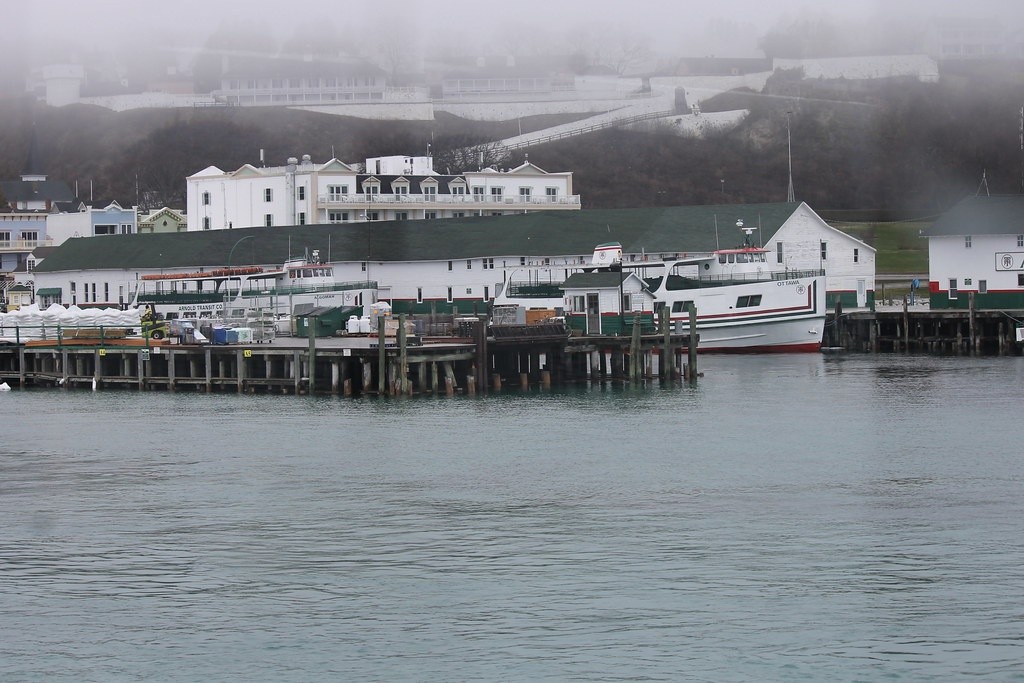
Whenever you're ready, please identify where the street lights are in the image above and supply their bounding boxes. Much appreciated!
[785,111,796,201]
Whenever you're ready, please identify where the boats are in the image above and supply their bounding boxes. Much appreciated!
[493,226,826,356]
[127,257,377,338]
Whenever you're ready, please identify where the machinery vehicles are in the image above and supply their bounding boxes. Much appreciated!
[128,302,166,340]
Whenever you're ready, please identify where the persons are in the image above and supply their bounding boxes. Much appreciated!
[617,251,621,257]
